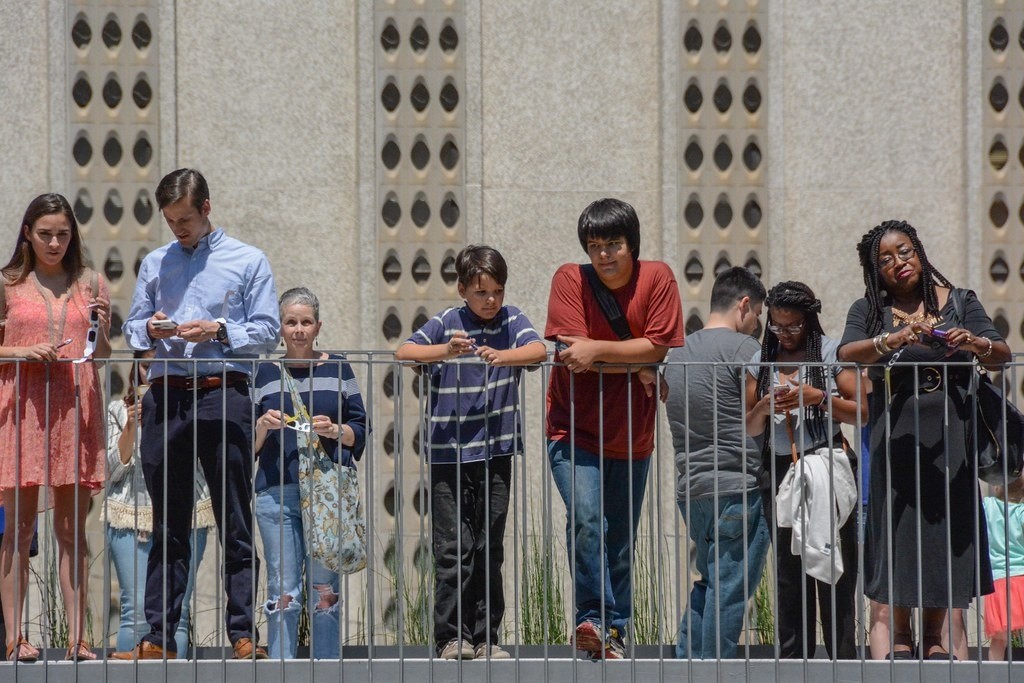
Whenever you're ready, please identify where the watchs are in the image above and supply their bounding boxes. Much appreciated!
[211,321,227,341]
[878,332,893,352]
[815,390,829,409]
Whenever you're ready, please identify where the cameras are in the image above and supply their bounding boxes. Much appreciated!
[921,329,962,351]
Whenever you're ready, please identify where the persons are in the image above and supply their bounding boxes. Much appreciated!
[395,244,548,659]
[0,194,112,662]
[247,287,373,659]
[834,362,1024,661]
[543,198,685,658]
[99,349,217,659]
[742,281,870,659]
[662,267,771,659]
[112,169,281,660]
[843,219,1014,660]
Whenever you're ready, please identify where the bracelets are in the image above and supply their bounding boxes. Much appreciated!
[978,337,992,358]
[333,424,344,439]
[873,335,885,357]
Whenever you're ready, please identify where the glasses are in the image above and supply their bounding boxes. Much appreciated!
[767,318,807,335]
[877,247,917,269]
[72,304,100,364]
[285,405,318,433]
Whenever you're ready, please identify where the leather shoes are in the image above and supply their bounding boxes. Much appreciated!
[108,642,177,660]
[232,637,269,658]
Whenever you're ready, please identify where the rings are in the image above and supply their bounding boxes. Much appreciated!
[966,333,971,343]
[908,334,916,340]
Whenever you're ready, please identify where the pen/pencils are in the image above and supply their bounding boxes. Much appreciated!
[57,339,73,348]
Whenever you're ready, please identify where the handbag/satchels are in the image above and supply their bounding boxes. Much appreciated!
[299,462,367,575]
[964,374,1024,485]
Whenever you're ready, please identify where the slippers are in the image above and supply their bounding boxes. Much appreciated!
[6,637,39,660]
[64,639,96,659]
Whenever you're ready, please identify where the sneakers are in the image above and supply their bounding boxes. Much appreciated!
[474,641,510,659]
[592,629,626,659]
[570,621,611,651]
[441,638,475,659]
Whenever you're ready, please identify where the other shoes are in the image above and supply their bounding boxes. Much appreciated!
[916,644,957,660]
[886,651,913,660]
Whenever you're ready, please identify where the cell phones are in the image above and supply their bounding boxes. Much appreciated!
[151,320,179,331]
[769,384,791,399]
